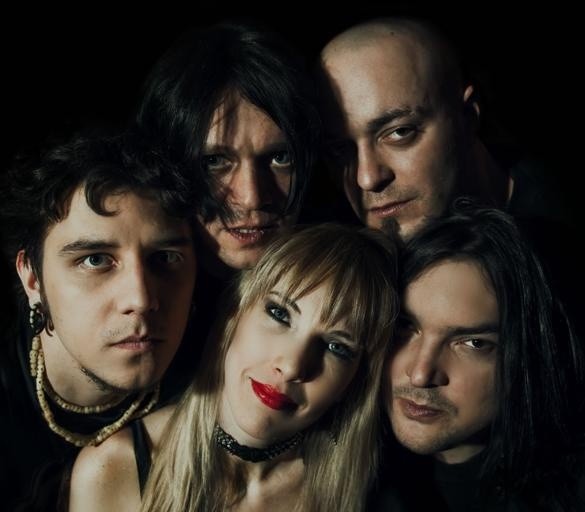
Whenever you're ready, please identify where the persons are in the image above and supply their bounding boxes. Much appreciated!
[128,29,345,270]
[62,213,406,510]
[2,117,238,511]
[369,199,585,511]
[311,16,524,247]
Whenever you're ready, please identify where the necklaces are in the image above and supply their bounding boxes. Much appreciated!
[27,334,162,449]
[207,424,308,462]
